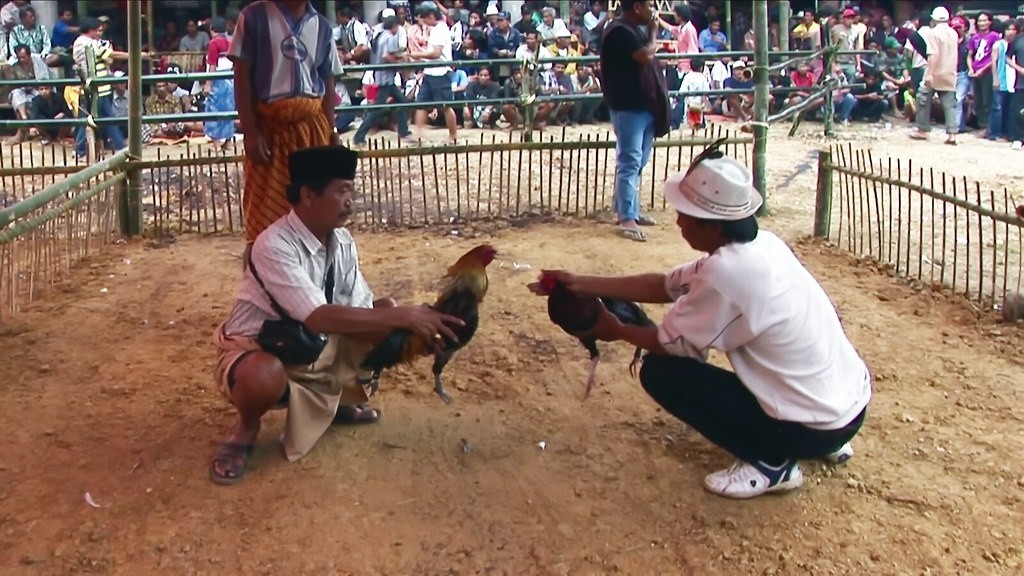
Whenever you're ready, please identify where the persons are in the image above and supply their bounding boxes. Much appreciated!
[602,1,673,243]
[0,0,1024,163]
[524,138,873,500]
[224,0,342,274]
[206,144,468,486]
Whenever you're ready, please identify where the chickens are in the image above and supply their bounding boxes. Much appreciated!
[543,272,657,404]
[360,245,502,406]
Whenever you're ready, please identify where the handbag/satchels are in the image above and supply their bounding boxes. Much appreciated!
[258,318,328,367]
[686,102,703,111]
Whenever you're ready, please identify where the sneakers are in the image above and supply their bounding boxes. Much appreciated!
[823,441,856,464]
[704,457,803,499]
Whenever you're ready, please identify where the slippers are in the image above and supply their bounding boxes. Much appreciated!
[332,404,382,423]
[636,214,658,226]
[209,441,253,484]
[619,224,648,242]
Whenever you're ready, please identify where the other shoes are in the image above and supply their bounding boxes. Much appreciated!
[910,131,1022,150]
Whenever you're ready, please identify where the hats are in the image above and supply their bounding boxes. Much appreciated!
[732,61,746,68]
[664,139,763,221]
[481,1,578,43]
[166,63,182,75]
[287,145,358,180]
[791,5,966,48]
[382,7,396,18]
[113,70,127,78]
